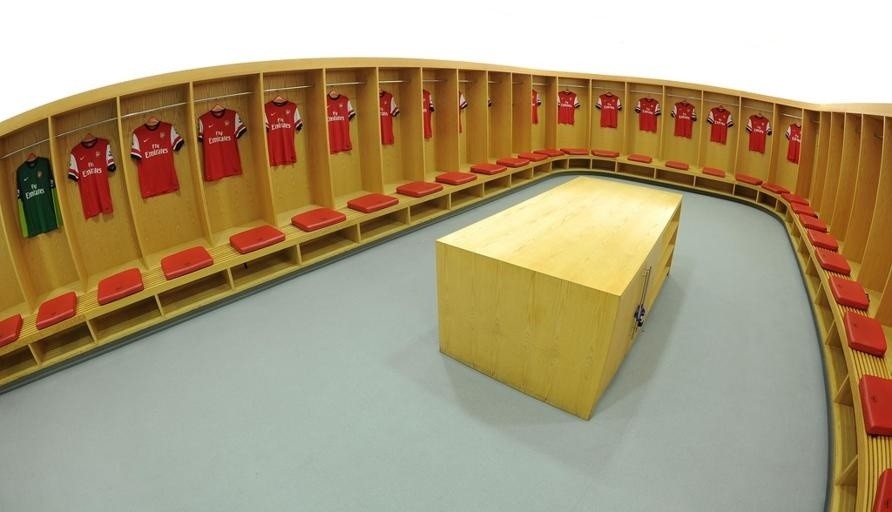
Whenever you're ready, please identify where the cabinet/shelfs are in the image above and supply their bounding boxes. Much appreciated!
[432,175,685,421]
